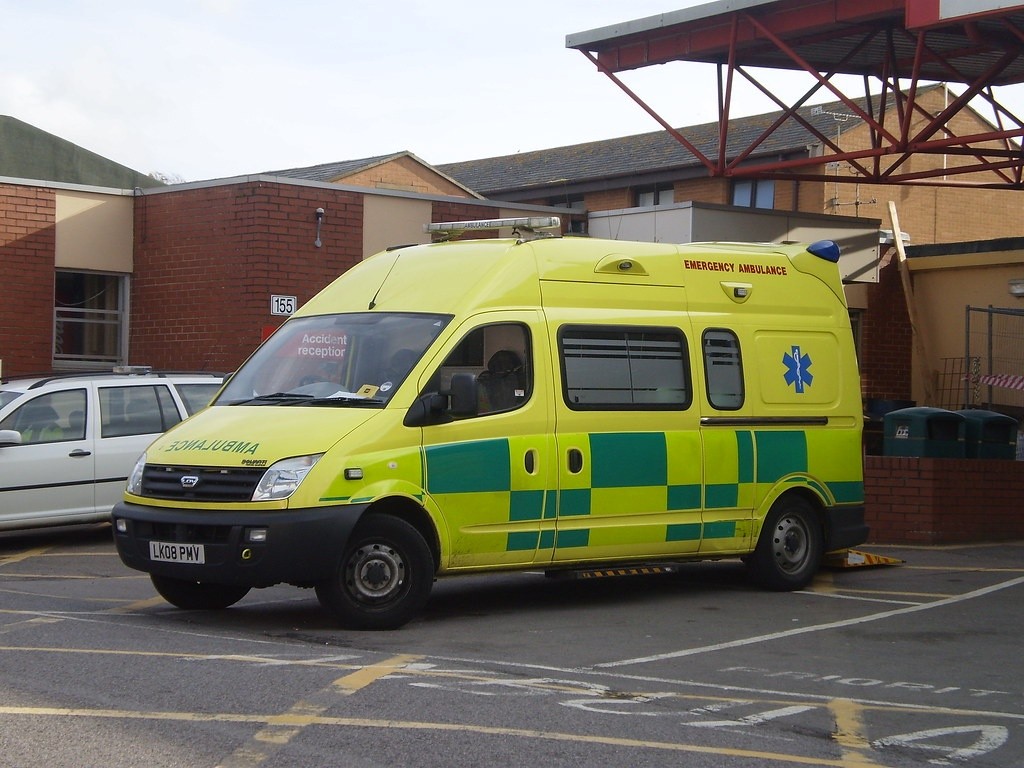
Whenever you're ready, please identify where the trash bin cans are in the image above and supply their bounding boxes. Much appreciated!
[882,407,967,457]
[957,409,1019,459]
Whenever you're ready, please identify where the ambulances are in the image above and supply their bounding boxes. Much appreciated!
[108,216,865,631]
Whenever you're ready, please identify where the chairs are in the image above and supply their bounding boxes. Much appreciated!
[472,350,524,414]
[64,407,84,439]
[376,348,419,399]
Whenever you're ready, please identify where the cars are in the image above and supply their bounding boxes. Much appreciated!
[0,366,260,535]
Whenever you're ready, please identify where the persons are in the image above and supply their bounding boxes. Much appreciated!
[20,402,63,443]
